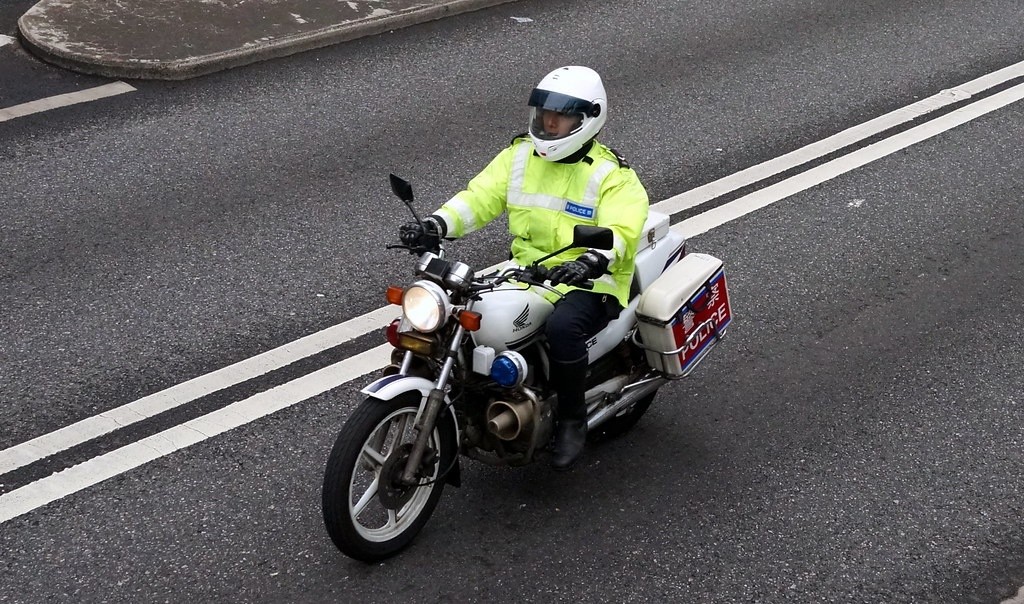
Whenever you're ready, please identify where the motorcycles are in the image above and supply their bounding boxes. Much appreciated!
[319,172,736,566]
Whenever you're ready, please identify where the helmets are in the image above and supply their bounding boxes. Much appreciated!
[528,65,608,162]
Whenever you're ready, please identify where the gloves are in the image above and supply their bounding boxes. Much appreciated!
[400,214,447,246]
[546,250,606,286]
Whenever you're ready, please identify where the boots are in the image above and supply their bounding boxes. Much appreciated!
[550,352,588,470]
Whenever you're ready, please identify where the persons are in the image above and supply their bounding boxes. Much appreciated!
[400,66,650,470]
